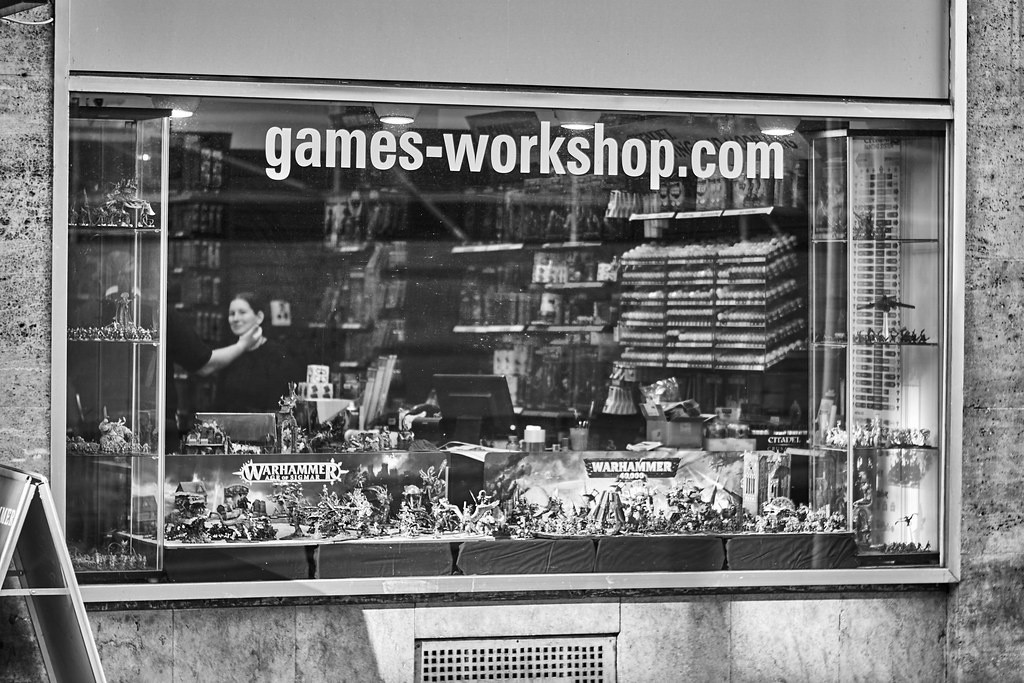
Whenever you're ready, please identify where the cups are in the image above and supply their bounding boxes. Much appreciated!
[522,441,547,453]
[570,428,589,451]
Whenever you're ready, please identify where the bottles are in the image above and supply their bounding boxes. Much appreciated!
[506,436,520,451]
[540,298,556,323]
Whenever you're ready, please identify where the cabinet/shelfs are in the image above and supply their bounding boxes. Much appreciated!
[807,128,943,565]
[171,132,805,372]
[66,107,171,573]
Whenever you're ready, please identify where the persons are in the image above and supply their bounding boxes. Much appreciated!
[68,251,293,451]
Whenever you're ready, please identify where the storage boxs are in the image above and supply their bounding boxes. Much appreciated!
[640,401,807,466]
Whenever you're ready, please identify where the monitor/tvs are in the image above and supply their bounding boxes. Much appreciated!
[433,373,519,445]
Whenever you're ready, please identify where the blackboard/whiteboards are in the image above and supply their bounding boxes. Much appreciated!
[0,463,104,683]
